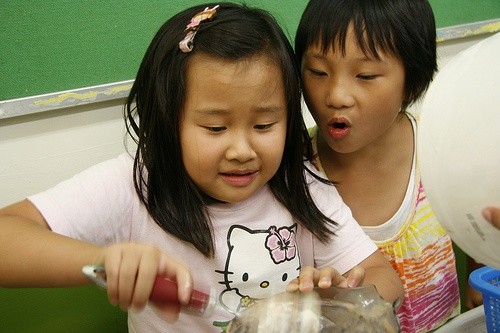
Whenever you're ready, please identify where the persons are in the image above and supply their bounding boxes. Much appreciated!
[1,0,405,333]
[282,0,487,333]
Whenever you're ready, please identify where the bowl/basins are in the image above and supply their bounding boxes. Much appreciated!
[225,282,400,333]
